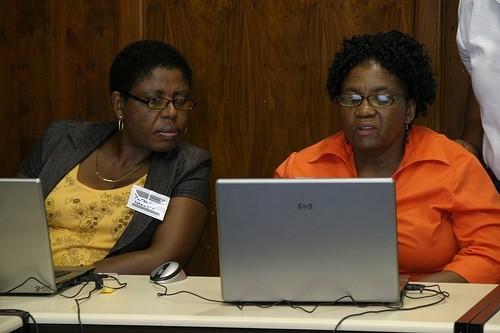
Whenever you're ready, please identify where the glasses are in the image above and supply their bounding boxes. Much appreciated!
[114,90,197,111]
[334,90,413,108]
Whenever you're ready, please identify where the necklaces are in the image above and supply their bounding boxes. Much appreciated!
[96,145,150,184]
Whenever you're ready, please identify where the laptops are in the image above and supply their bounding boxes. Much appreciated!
[214,178,411,303]
[0,178,96,294]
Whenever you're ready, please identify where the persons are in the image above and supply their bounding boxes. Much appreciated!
[456,0,500,191]
[272,30,500,287]
[18,41,213,275]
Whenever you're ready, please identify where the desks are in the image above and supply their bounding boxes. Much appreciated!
[0,273,500,333]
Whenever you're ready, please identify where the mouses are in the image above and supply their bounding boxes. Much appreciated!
[149,261,187,284]
